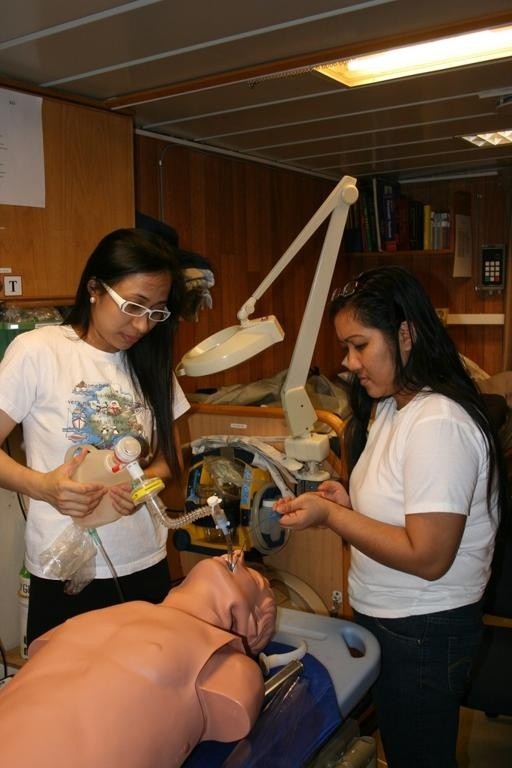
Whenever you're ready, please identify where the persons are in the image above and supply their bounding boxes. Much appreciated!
[0,225,192,656]
[276,267,511,767]
[0,550,279,767]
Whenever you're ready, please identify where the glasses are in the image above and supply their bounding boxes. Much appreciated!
[329,281,360,301]
[102,281,170,322]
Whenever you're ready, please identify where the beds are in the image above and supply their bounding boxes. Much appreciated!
[184,607,391,764]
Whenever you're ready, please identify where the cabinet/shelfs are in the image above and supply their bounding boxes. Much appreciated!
[346,247,508,327]
[0,87,138,314]
